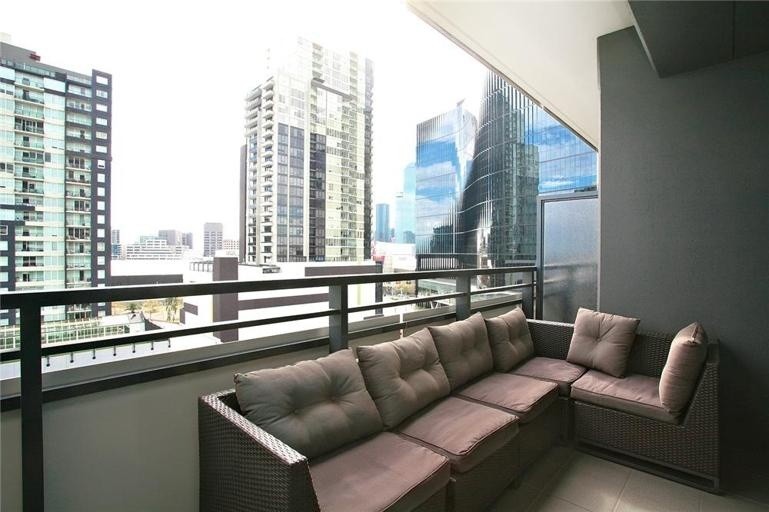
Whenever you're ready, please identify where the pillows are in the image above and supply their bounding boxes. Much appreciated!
[234,345,385,461]
[355,326,453,430]
[565,306,641,379]
[485,306,536,373]
[659,320,710,420]
[429,309,494,393]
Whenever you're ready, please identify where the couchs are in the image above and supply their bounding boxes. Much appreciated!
[198,319,722,512]
